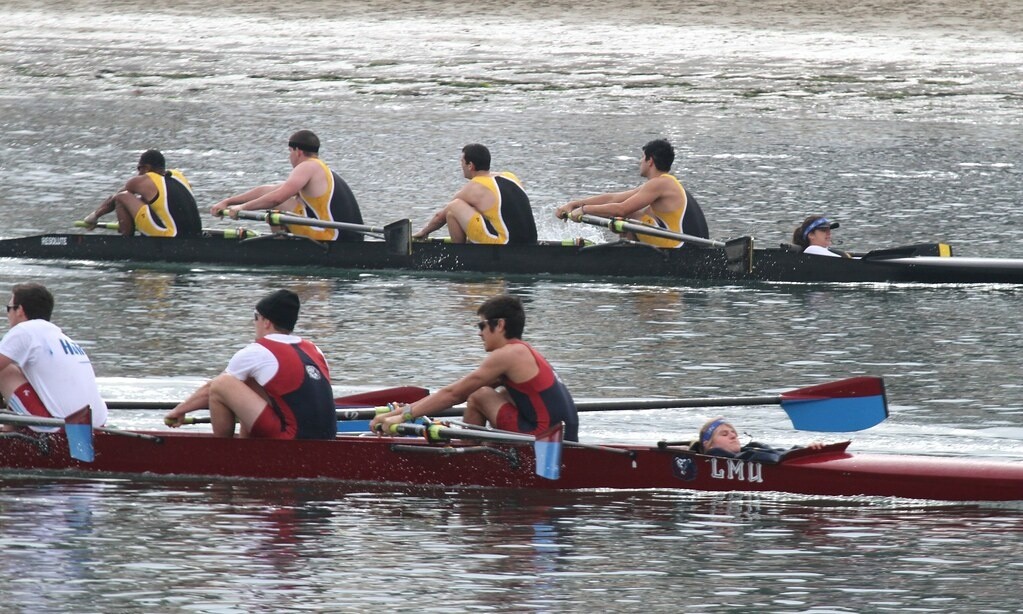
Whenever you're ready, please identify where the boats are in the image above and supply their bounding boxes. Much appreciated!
[0,226,1023,287]
[0,416,1023,500]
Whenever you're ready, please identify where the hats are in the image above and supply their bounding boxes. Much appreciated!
[804,218,840,246]
[256,289,300,330]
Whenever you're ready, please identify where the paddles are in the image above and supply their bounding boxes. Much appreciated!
[778,241,954,261]
[217,209,413,257]
[0,404,95,463]
[414,238,576,249]
[163,376,889,433]
[104,386,430,410]
[74,220,273,240]
[561,212,754,274]
[374,421,566,481]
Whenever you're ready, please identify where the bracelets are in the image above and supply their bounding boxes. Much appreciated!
[582,203,586,214]
[403,408,413,420]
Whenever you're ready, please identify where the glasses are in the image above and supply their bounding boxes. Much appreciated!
[6,305,18,311]
[815,226,834,232]
[137,165,144,170]
[254,311,263,321]
[477,318,505,331]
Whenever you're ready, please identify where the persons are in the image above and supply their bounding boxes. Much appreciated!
[369,295,579,442]
[164,288,337,440]
[210,129,364,241]
[555,140,709,249]
[793,215,840,257]
[84,150,202,237]
[412,143,538,245]
[690,416,825,462]
[0,282,107,433]
